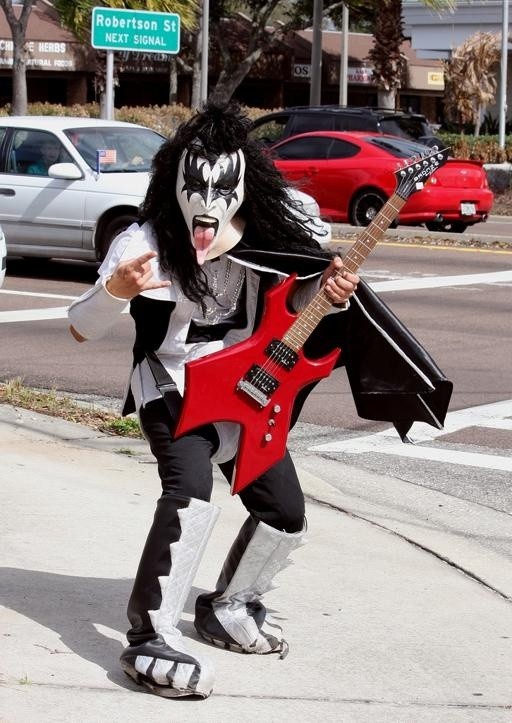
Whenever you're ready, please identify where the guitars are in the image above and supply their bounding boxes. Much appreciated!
[174,146,451,497]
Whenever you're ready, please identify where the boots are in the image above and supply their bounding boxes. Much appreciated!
[191,514,309,658]
[119,492,223,701]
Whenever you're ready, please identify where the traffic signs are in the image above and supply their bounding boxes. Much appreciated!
[91,7,184,56]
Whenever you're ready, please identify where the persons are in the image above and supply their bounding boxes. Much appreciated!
[27,135,62,175]
[67,104,359,699]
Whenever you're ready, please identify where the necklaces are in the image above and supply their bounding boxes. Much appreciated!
[184,261,248,323]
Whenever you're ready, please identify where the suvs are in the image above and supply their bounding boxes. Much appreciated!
[220,97,447,180]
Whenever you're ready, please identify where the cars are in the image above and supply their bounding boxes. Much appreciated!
[1,108,333,289]
[249,126,495,235]
